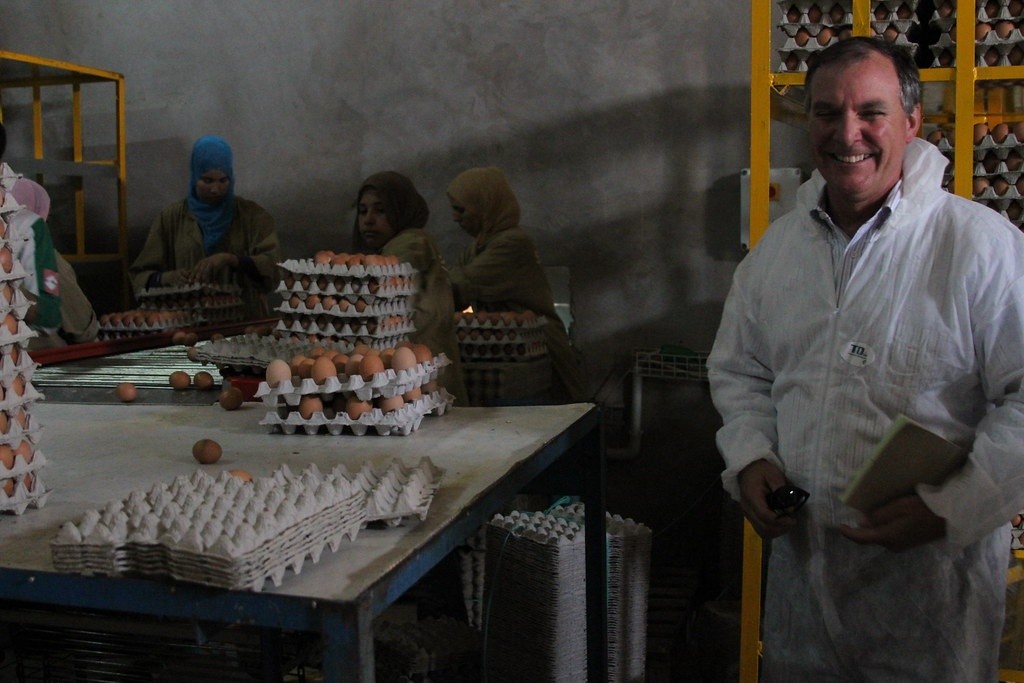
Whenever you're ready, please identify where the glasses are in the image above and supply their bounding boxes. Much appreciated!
[767,486,810,516]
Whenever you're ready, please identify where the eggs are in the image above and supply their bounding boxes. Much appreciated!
[93,282,246,334]
[783,0,1022,229]
[169,347,243,410]
[192,438,222,464]
[117,383,136,401]
[1011,515,1024,550]
[172,326,271,345]
[228,471,252,483]
[264,251,538,419]
[0,185,42,514]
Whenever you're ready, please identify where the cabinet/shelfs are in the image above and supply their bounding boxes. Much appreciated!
[0,50,130,310]
[749,0,1024,251]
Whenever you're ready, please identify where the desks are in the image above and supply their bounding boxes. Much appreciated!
[0,403,609,682]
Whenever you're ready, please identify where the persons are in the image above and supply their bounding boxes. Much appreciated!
[0,122,63,329]
[442,165,579,396]
[11,177,105,350]
[130,135,282,301]
[351,171,472,408]
[706,38,1024,683]
